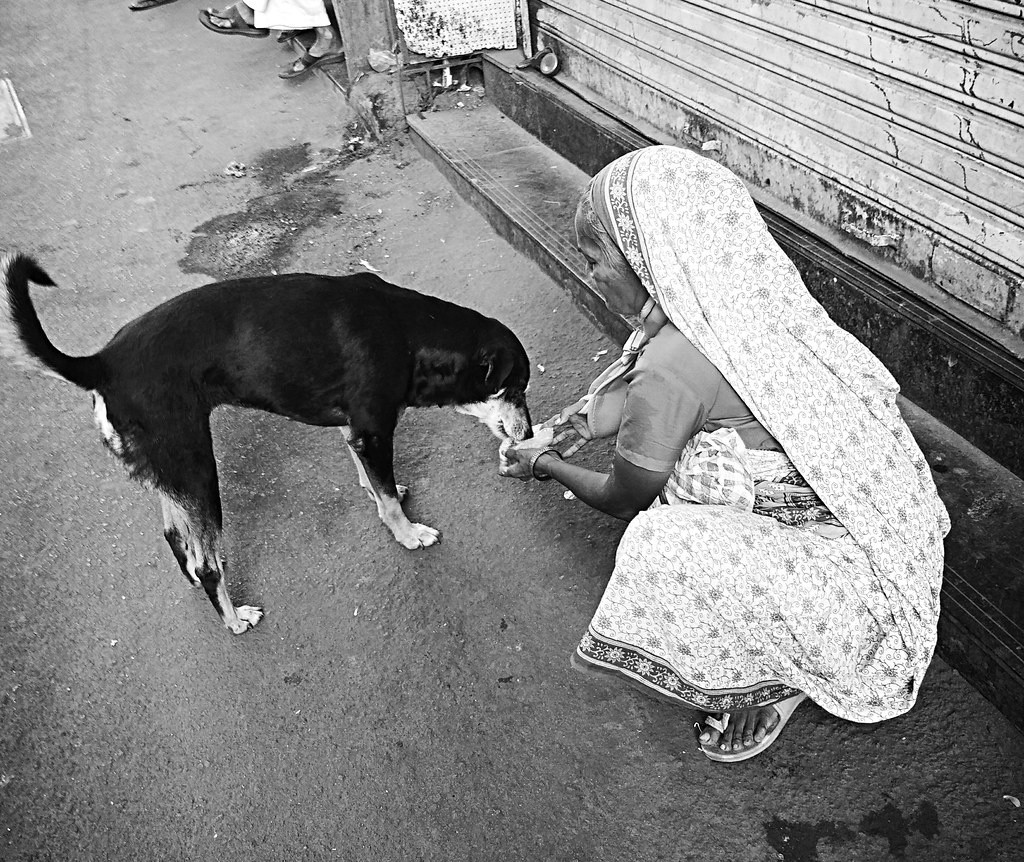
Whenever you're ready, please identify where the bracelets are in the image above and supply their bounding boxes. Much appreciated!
[529,447,564,483]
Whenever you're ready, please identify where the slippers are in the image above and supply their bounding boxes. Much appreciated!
[279,47,346,78]
[198,4,271,38]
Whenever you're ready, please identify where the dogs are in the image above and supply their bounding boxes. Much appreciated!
[0,248,535,639]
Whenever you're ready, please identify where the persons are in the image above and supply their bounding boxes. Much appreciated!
[484,145,951,768]
[128,0,345,81]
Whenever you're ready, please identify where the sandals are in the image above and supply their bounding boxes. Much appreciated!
[698,693,810,763]
